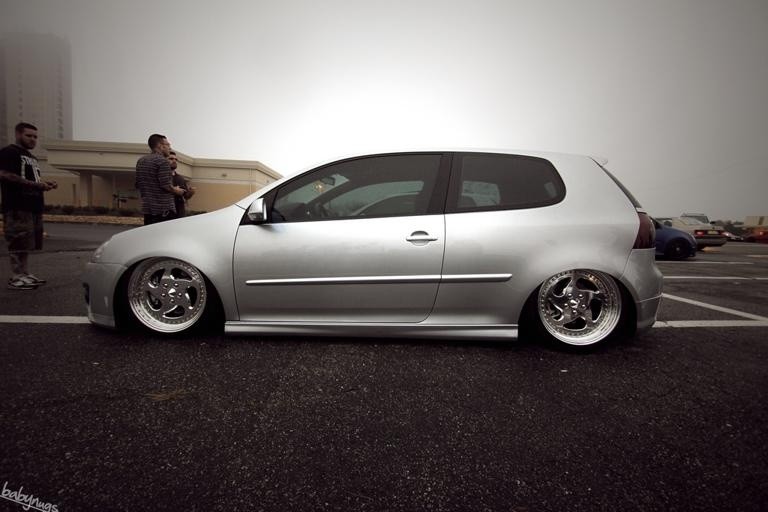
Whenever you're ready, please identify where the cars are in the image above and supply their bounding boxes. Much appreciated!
[80,146,665,355]
[647,211,768,261]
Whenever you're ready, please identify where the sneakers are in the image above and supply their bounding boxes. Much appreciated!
[7,272,48,291]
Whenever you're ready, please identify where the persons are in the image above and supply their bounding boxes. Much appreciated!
[164,152,195,218]
[135,134,185,224]
[0,123,57,290]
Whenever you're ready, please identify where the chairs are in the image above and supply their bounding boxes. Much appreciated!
[409,174,552,212]
